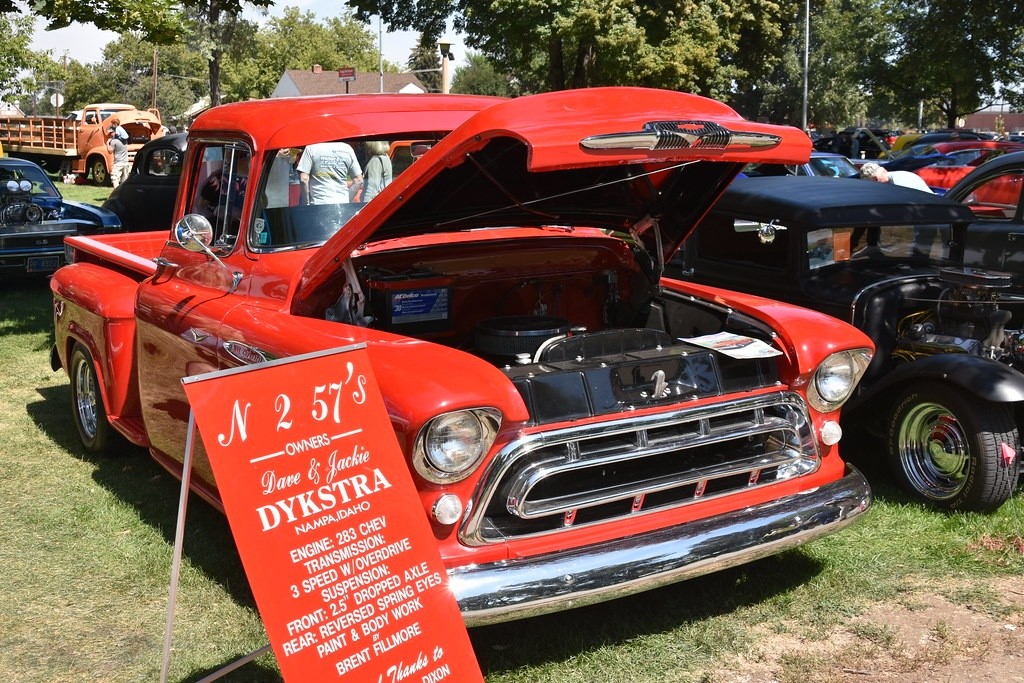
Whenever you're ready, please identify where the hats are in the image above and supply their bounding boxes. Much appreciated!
[860,162,879,179]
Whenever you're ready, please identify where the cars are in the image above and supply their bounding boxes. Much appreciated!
[0,127,1024,511]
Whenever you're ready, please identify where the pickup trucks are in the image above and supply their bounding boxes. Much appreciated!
[47,85,876,630]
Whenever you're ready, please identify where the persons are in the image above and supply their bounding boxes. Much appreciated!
[297,142,364,205]
[359,141,393,203]
[861,162,936,195]
[106,127,129,188]
[110,118,129,140]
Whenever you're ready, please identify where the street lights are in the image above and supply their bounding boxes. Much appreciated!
[435,41,455,92]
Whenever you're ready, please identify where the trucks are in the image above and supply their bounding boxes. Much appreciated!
[0,103,166,186]
